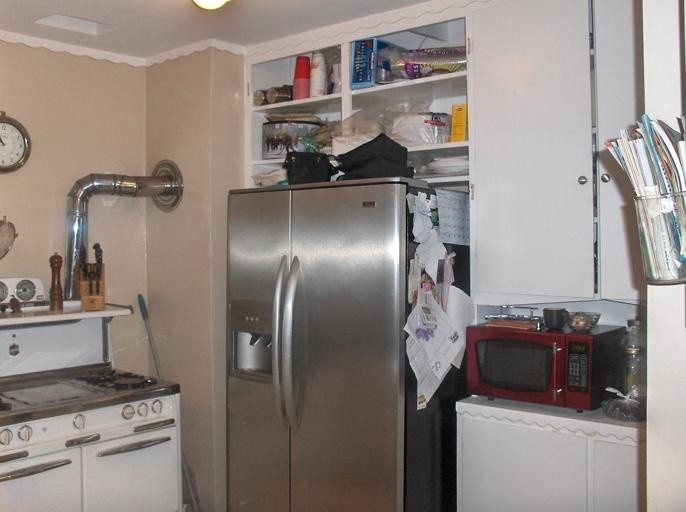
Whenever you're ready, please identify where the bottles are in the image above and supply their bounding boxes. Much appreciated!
[620,343,647,402]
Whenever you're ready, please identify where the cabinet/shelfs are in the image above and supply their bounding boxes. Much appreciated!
[470,0,643,319]
[249,15,468,189]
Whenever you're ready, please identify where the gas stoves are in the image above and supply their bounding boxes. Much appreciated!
[0,360,180,426]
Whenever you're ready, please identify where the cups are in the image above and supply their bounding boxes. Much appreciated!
[543,306,570,330]
[292,52,327,104]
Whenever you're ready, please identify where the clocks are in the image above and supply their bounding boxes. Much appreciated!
[1,111,31,172]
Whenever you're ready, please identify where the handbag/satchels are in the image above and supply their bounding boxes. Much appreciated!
[281,144,339,185]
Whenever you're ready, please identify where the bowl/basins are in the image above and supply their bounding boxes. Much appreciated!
[566,310,602,333]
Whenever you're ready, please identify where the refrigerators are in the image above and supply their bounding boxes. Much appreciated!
[224,176,469,511]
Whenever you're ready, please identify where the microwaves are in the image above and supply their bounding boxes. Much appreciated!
[463,313,628,412]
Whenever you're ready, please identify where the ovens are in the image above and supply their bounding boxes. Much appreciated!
[0,394,182,512]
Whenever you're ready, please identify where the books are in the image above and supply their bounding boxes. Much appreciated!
[603,112,686,284]
